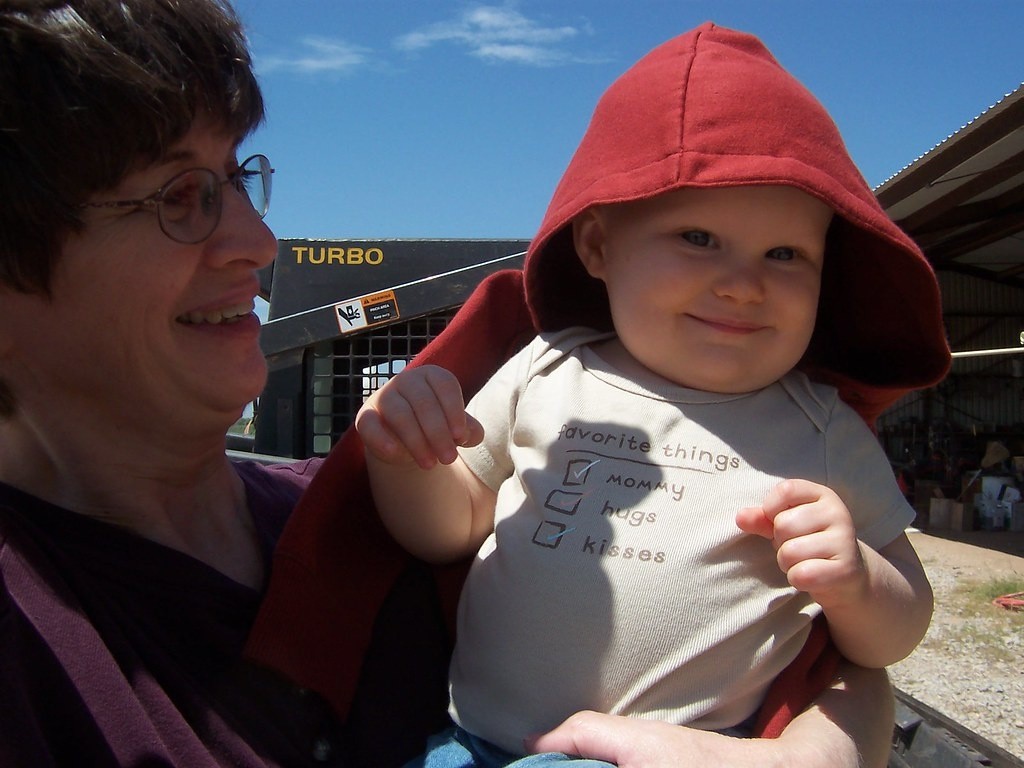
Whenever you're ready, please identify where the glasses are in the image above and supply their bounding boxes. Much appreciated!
[76,154,277,243]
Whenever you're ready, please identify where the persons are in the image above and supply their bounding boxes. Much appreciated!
[0,0,894,768]
[356,22,952,768]
